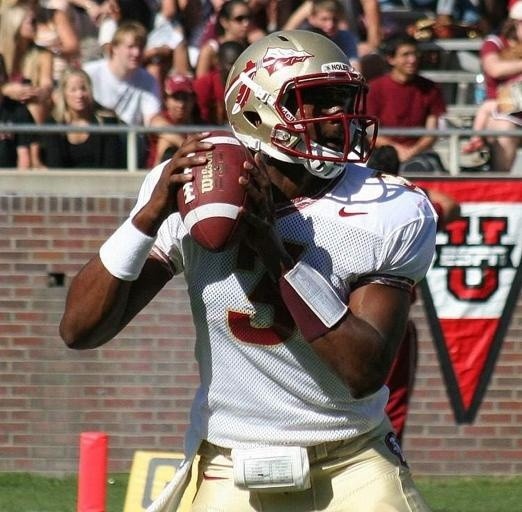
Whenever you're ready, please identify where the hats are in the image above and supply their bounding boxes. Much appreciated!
[162,74,197,96]
[506,1,522,23]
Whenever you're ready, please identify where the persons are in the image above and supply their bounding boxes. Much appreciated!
[60,30,439,511]
[366,145,454,452]
[0,0,522,174]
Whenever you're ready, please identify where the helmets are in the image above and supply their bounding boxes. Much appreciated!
[220,26,367,157]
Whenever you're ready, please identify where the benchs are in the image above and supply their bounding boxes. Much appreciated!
[351,1,522,176]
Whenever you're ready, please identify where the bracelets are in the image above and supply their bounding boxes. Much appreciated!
[275,259,349,344]
[99,217,157,282]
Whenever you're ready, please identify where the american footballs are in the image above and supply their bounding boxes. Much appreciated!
[178,130,254,252]
